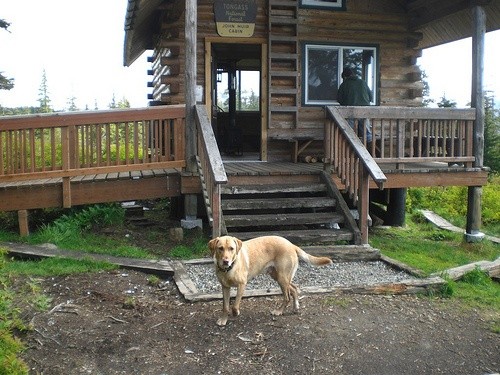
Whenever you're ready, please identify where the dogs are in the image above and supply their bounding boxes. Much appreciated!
[207,235,332,326]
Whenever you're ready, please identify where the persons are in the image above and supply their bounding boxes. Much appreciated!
[337,69,372,153]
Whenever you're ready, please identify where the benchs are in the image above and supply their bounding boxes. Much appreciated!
[270,132,397,164]
